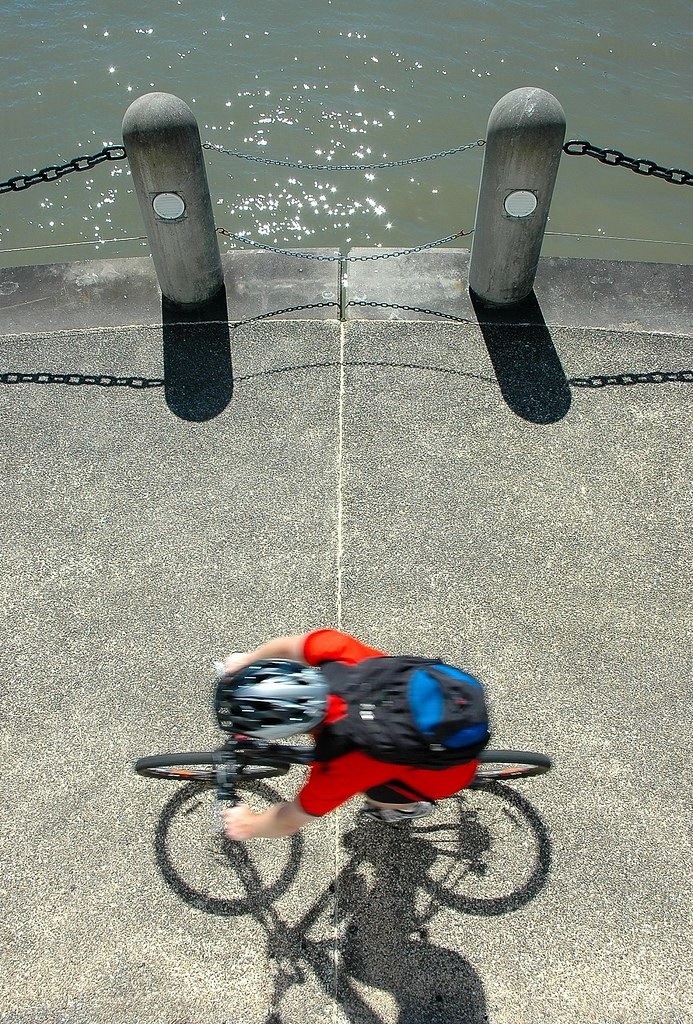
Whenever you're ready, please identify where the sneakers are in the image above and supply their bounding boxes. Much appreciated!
[366,800,433,822]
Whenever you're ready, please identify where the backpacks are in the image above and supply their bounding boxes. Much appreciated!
[314,656,490,770]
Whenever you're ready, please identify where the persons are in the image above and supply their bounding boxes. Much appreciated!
[220,631,482,841]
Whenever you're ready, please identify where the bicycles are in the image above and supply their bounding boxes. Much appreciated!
[131,649,553,826]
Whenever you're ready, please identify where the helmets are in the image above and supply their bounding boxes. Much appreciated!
[215,658,329,740]
